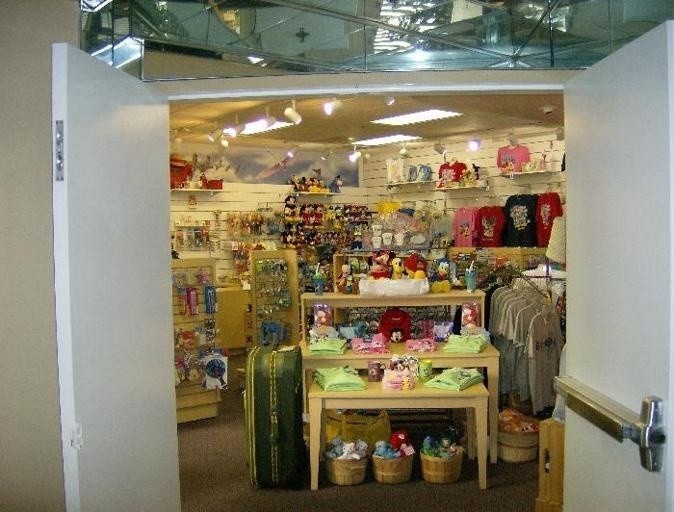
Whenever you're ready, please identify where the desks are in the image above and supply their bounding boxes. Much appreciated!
[333,252,457,326]
[301,289,487,337]
[299,334,501,467]
[307,371,490,491]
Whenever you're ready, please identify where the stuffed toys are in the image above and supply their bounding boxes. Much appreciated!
[374,429,416,457]
[462,307,476,328]
[324,438,369,461]
[283,175,368,246]
[316,306,329,327]
[423,436,457,458]
[500,409,539,432]
[337,249,452,294]
[390,328,403,343]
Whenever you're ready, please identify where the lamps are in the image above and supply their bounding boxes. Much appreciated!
[283,99,303,125]
[209,123,224,143]
[349,145,361,163]
[324,97,344,115]
[257,105,276,129]
[434,143,447,156]
[221,139,230,147]
[230,113,246,138]
[287,145,298,158]
[400,150,409,158]
[383,96,395,107]
[468,139,481,151]
[320,149,331,160]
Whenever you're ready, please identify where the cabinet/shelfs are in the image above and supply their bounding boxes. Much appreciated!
[172,257,222,423]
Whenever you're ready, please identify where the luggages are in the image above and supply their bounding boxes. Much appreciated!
[243,341,303,486]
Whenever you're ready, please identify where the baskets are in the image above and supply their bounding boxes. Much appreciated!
[371,452,413,484]
[324,452,368,486]
[419,446,464,484]
[497,414,542,464]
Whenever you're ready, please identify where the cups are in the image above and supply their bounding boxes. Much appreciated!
[465,271,477,292]
[368,360,386,381]
[419,359,432,381]
[313,279,323,296]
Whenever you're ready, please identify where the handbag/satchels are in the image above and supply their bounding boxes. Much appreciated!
[304,409,391,452]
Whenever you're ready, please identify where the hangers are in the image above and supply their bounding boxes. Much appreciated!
[509,274,554,316]
